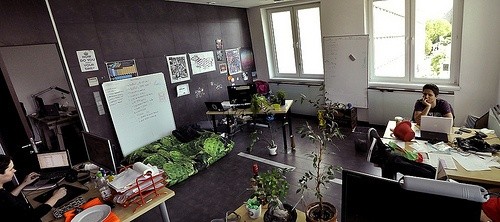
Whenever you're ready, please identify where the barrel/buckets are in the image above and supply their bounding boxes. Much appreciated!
[393,122,415,142]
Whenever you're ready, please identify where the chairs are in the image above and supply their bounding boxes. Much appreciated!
[254,80,272,141]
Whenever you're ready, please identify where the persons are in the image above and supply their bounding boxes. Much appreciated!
[367,128,500,222]
[414,84,453,127]
[0,154,67,222]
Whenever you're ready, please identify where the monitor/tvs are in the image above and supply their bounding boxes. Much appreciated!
[82,131,117,177]
[341,169,482,222]
[227,85,256,104]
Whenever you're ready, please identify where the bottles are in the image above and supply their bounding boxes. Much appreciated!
[252,164,266,203]
[96,172,115,209]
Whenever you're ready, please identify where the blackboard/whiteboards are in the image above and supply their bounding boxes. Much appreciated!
[323,34,369,109]
[101,72,176,157]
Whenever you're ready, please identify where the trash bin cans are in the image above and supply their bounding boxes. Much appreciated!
[352,126,369,146]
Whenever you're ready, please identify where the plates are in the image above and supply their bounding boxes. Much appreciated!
[69,204,111,222]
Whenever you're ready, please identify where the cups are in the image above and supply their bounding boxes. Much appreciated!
[246,203,261,219]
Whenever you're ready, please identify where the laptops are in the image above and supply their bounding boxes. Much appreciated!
[420,116,453,134]
[204,101,230,111]
[23,149,72,190]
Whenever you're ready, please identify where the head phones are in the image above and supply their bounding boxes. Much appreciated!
[64,168,90,183]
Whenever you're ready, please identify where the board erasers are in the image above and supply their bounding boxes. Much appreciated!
[349,55,355,61]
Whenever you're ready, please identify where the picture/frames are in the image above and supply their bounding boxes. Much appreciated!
[225,47,243,76]
[218,63,228,75]
[188,50,216,74]
[165,53,191,84]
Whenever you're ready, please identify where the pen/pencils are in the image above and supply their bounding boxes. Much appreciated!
[56,183,59,190]
[417,100,423,102]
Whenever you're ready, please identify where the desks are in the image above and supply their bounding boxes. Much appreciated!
[29,107,80,152]
[205,99,294,150]
[228,202,306,222]
[15,162,174,222]
[375,119,500,185]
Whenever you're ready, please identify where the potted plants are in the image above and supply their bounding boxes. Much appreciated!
[245,197,261,219]
[253,170,297,222]
[298,83,348,222]
[246,111,278,156]
[277,90,287,106]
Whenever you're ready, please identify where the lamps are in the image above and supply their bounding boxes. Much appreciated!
[29,86,70,105]
